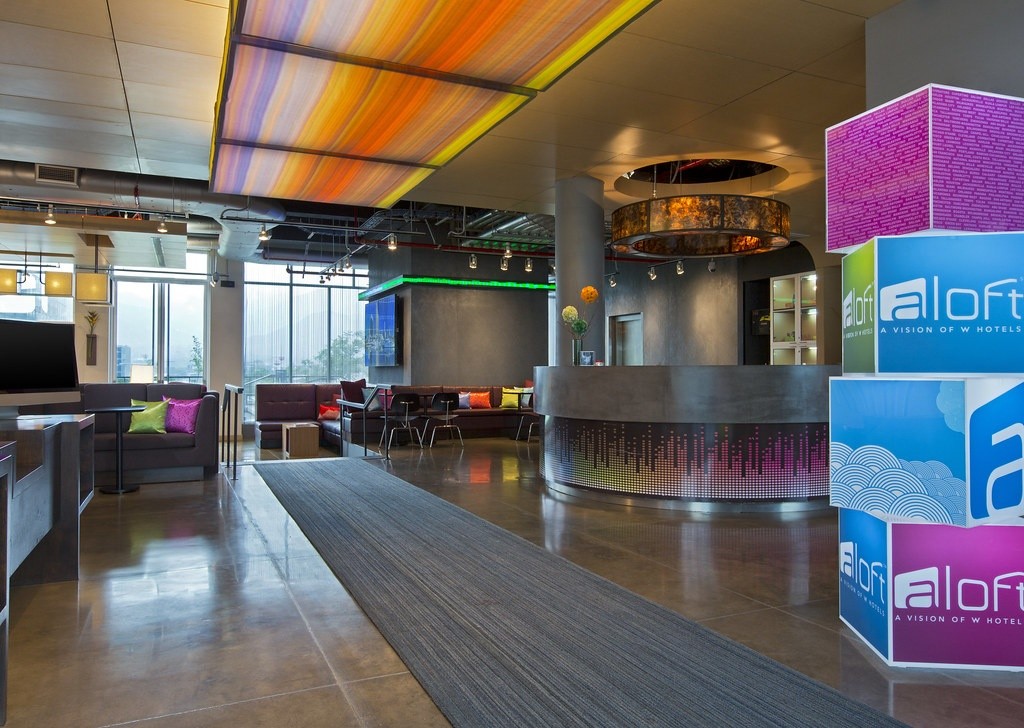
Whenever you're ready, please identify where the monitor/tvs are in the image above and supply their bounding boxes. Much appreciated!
[0,319,81,420]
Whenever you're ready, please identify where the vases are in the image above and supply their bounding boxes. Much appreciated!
[572,339,583,366]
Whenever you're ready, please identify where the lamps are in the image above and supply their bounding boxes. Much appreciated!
[45,206,56,225]
[84,406,144,494]
[469,241,556,273]
[320,255,351,283]
[387,233,398,251]
[0,231,114,306]
[608,162,791,286]
[209,272,220,288]
[157,215,168,233]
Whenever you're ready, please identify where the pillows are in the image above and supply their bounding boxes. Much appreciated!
[129,396,202,434]
[317,380,535,420]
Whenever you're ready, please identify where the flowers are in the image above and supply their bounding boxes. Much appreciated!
[562,286,599,338]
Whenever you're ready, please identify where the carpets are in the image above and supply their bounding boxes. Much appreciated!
[252,457,915,728]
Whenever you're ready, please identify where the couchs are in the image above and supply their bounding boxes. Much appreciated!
[18,383,221,486]
[255,382,372,448]
[391,384,539,439]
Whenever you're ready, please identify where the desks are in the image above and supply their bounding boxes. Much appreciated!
[0,413,97,727]
[503,392,533,438]
[379,394,435,446]
[282,424,320,459]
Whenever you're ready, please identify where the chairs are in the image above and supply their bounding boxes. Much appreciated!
[378,392,423,449]
[516,412,540,443]
[419,393,464,448]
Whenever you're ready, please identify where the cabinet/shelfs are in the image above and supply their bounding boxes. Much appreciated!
[769,271,817,367]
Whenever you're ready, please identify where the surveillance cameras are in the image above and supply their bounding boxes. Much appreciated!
[213,272,220,282]
[708,262,717,272]
